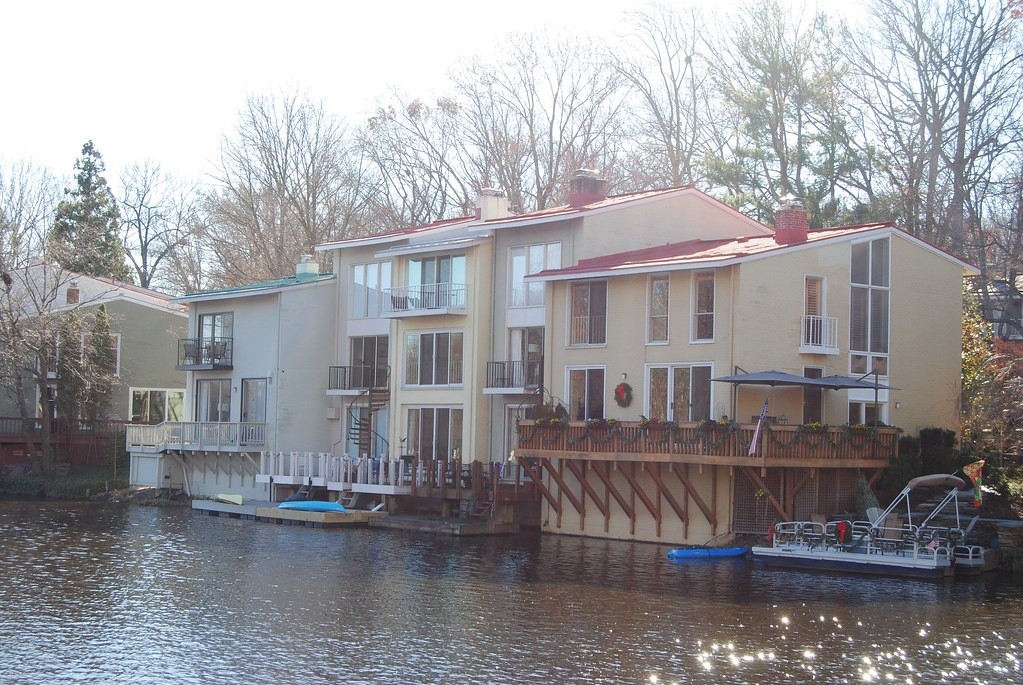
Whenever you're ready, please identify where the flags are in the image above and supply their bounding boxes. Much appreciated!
[749,400,768,456]
[928,534,939,549]
[964,461,985,508]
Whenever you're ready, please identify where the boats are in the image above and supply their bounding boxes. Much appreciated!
[750,473,986,582]
[666,546,750,559]
[216,494,244,505]
[278,501,348,513]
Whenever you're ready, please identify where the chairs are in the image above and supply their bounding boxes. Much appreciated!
[207,342,228,364]
[182,344,201,362]
[874,518,906,558]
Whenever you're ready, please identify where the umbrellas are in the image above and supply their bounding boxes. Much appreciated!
[707,365,902,427]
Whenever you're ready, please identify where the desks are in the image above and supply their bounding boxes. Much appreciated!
[874,538,906,554]
